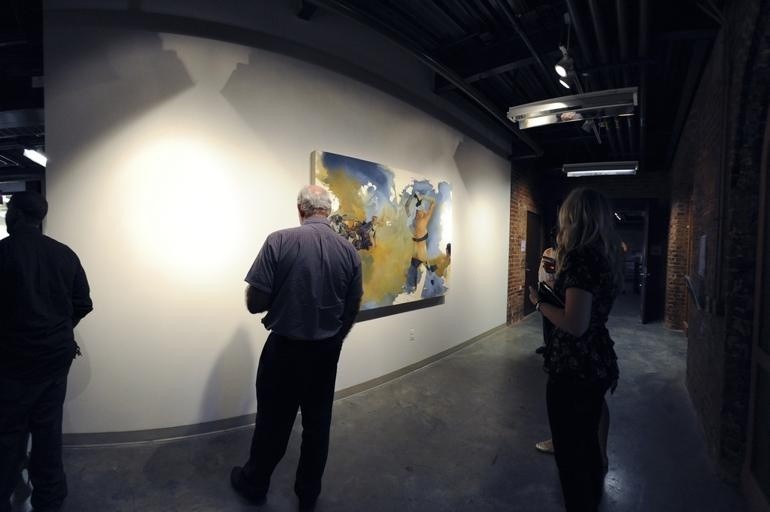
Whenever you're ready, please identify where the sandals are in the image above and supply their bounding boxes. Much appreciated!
[536,439,555,454]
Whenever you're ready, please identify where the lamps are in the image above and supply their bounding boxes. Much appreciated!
[509,47,640,130]
[561,159,640,180]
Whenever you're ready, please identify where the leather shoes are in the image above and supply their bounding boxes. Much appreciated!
[231,466,266,505]
[536,345,546,353]
[295,481,316,512]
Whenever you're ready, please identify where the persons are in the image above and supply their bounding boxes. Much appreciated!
[529,188,620,512]
[0,192,93,512]
[230,184,363,512]
[329,193,451,278]
